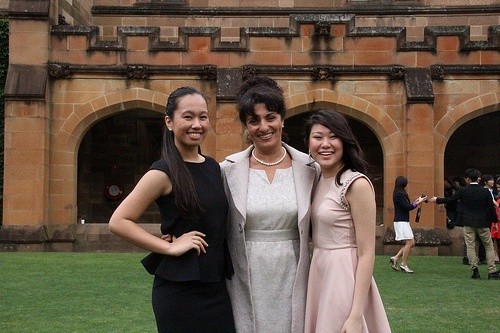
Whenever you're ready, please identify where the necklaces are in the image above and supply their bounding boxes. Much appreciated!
[252,146,286,166]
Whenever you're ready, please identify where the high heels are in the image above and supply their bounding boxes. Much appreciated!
[399,263,413,273]
[388,256,399,271]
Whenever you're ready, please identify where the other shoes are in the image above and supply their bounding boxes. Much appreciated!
[488,271,500,279]
[471,268,481,280]
[462,256,471,265]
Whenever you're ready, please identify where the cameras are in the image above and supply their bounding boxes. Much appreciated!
[421,193,429,203]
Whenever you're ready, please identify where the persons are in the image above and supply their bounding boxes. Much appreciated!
[387,176,428,273]
[161,76,337,333]
[429,169,500,279]
[109,86,236,333]
[304,110,390,333]
